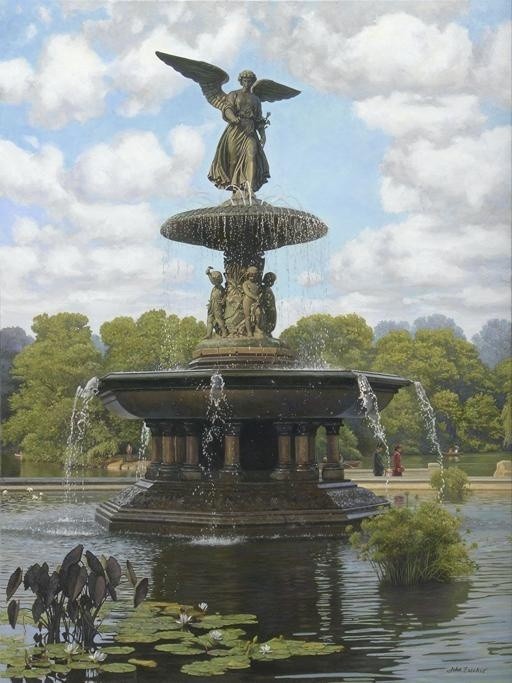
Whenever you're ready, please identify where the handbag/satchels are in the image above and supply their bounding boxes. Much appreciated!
[396,466,404,472]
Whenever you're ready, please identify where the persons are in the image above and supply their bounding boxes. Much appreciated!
[242,265,264,336]
[207,69,271,204]
[261,271,277,337]
[201,266,228,339]
[392,444,405,475]
[373,441,386,476]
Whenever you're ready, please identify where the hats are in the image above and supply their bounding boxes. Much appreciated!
[395,444,402,448]
